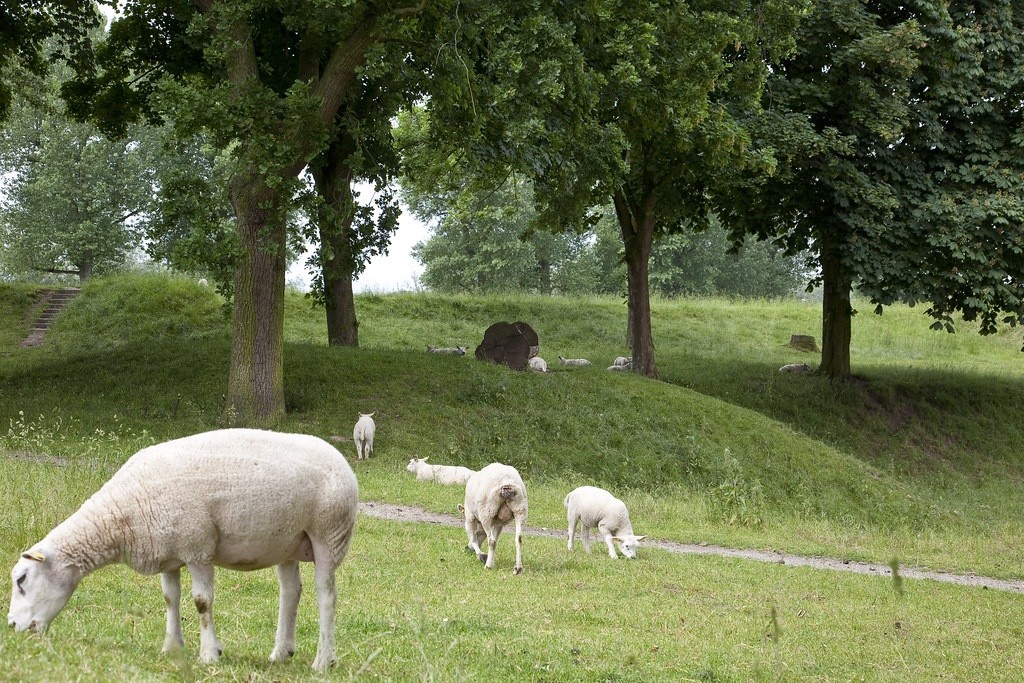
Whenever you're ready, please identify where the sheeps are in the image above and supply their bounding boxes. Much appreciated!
[405,453,528,575]
[606,356,633,370]
[559,356,592,365]
[564,486,646,560]
[425,345,469,357]
[353,411,377,461]
[7,427,360,667]
[197,279,208,287]
[528,357,548,372]
[778,363,814,373]
